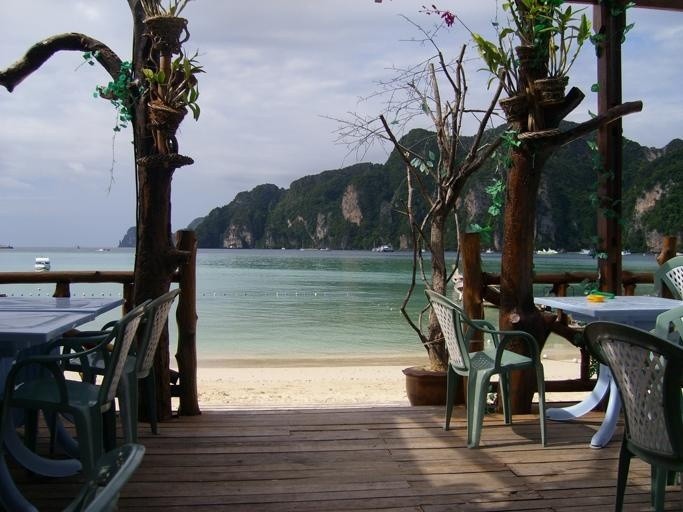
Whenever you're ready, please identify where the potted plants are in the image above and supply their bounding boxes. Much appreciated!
[137,0,189,52]
[499,1,593,103]
[141,47,206,137]
[317,12,514,406]
[470,28,527,122]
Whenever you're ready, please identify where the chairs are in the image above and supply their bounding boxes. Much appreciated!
[653,305,683,350]
[424,289,547,449]
[583,322,683,512]
[650,254,683,302]
[533,294,683,450]
[0,443,146,512]
[58,288,181,444]
[0,299,154,480]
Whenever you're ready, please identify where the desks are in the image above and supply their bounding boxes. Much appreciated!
[0,311,96,512]
[0,297,126,317]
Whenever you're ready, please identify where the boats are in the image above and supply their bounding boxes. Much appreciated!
[372,243,395,253]
[33,255,50,272]
[536,247,560,256]
